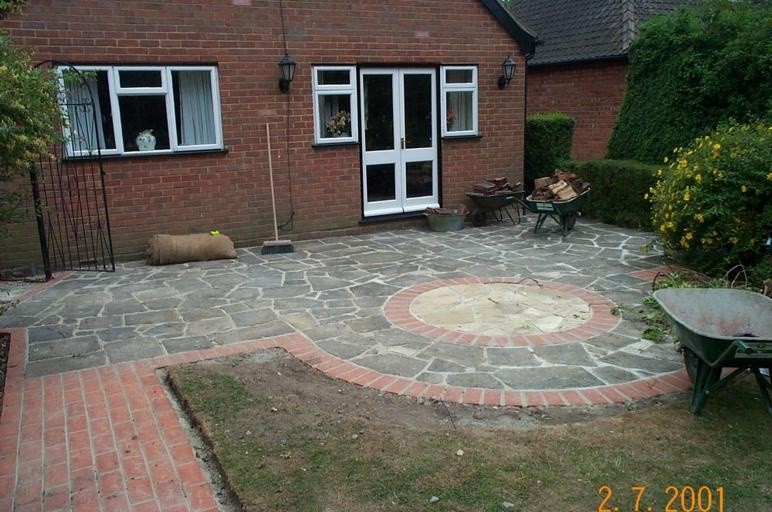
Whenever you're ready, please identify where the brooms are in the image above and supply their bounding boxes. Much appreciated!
[261,123,294,255]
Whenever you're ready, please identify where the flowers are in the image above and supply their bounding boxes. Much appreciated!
[447,112,455,129]
[325,110,352,135]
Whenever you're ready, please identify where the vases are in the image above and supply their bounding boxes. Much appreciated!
[136,133,157,150]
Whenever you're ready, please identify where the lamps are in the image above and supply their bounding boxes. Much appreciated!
[498,55,516,89]
[278,53,297,93]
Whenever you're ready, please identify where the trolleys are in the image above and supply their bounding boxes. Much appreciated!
[464,190,525,226]
[651,287,772,416]
[506,183,592,237]
